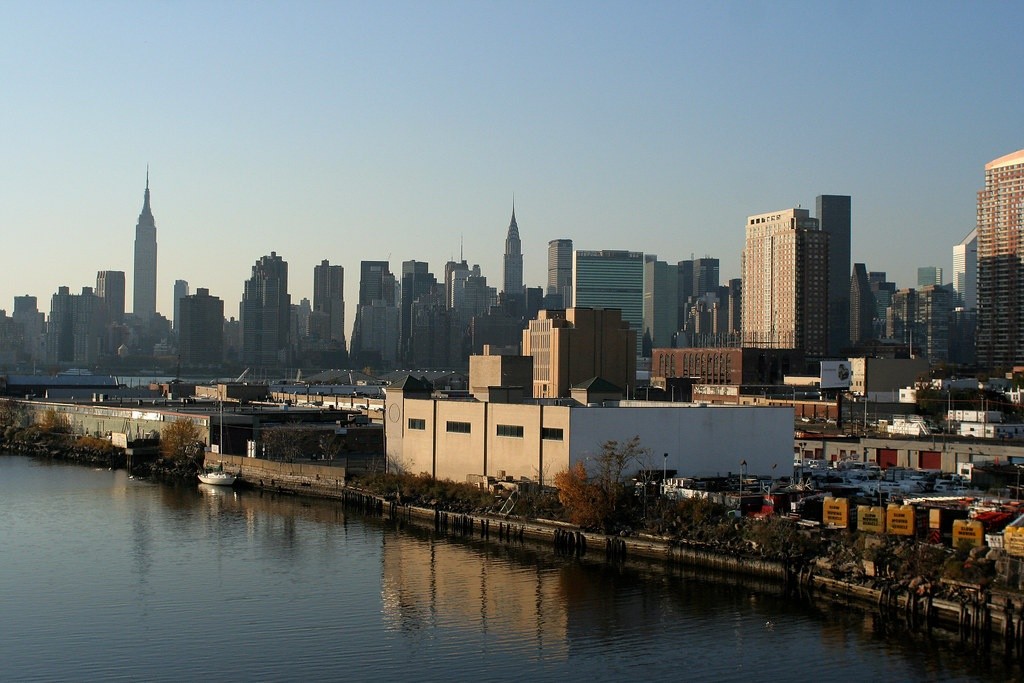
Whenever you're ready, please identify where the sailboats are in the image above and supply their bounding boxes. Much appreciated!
[197,395,237,485]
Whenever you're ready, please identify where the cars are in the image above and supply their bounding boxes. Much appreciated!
[802,415,880,428]
[725,455,971,502]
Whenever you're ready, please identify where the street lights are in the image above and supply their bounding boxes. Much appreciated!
[792,386,795,407]
[980,394,984,411]
[946,388,952,434]
[761,387,768,404]
[798,442,808,474]
[740,459,747,496]
[877,486,883,530]
[625,382,629,400]
[671,385,675,402]
[1014,463,1024,502]
[663,453,669,479]
